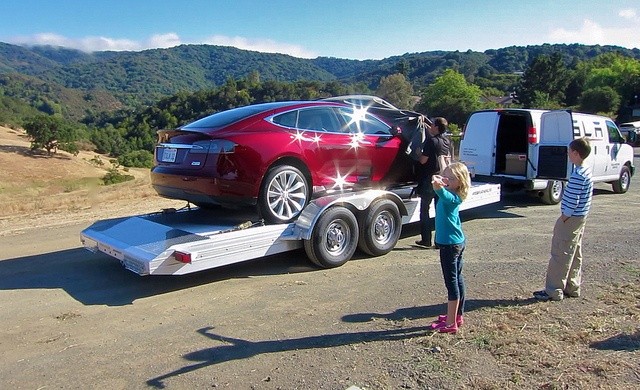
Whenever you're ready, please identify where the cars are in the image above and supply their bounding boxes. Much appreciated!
[150,101,434,224]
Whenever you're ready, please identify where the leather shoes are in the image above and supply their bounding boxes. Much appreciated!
[415,240,431,248]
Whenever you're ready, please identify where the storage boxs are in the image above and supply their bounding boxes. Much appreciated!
[506,153,527,175]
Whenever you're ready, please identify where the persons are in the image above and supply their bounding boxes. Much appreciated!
[415,117,449,248]
[431,163,470,333]
[533,136,593,299]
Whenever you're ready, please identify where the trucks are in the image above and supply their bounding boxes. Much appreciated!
[459,108,635,204]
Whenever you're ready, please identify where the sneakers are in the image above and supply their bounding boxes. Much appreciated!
[432,321,458,333]
[439,314,463,326]
[533,290,554,300]
